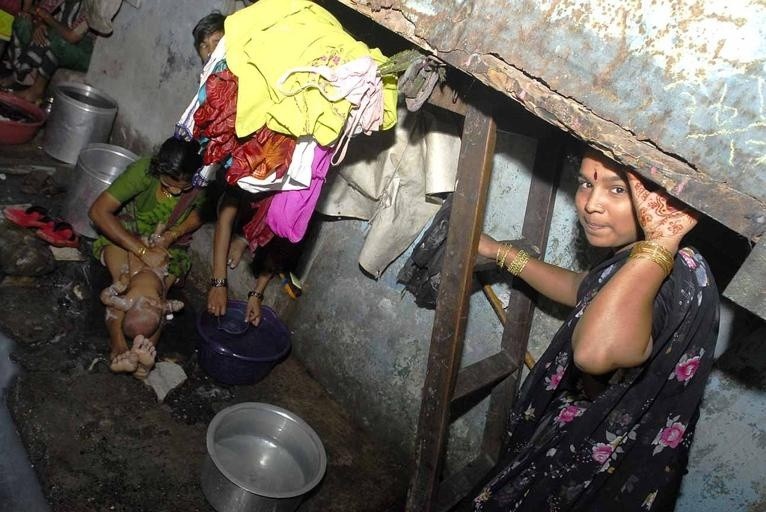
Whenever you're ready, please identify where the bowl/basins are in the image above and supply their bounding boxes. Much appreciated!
[0,91,46,144]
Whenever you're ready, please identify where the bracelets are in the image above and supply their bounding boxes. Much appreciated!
[630,242,675,275]
[493,242,529,277]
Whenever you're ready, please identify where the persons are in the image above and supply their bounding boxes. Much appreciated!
[88,11,291,378]
[467,152,719,510]
[1,0,102,102]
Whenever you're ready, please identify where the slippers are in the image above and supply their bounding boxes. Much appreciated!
[36,220,79,247]
[3,206,62,227]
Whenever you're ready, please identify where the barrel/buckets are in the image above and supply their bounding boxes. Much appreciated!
[44,80,117,169]
[63,143,143,242]
